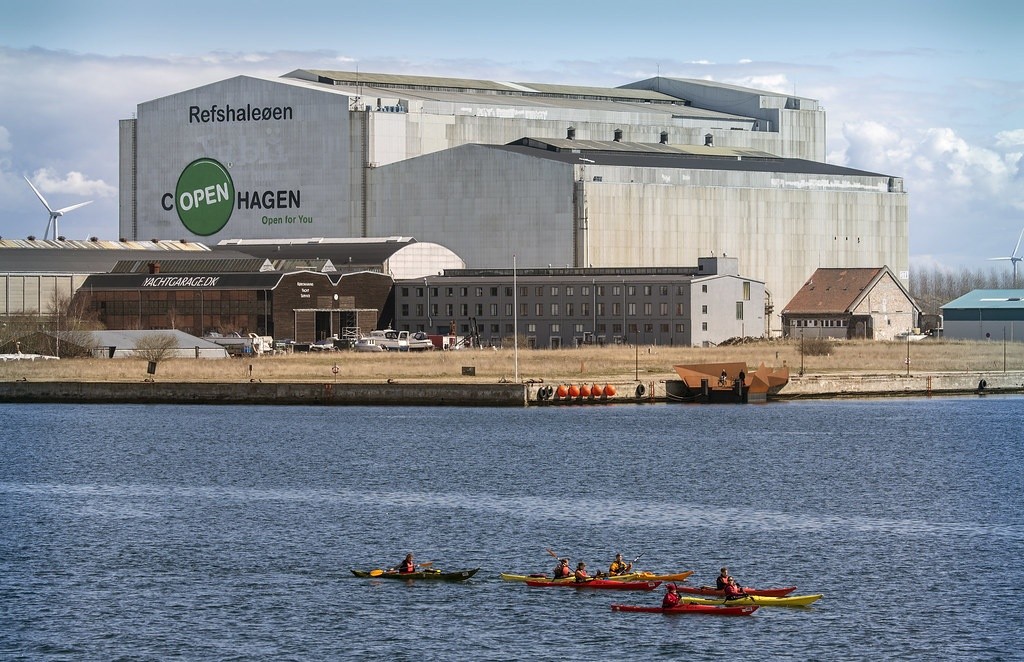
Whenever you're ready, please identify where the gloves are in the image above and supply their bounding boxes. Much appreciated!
[678,595,682,599]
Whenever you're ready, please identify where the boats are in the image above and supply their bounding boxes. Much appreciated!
[610,601,761,617]
[526,578,663,593]
[666,580,798,598]
[353,327,434,353]
[500,570,694,581]
[677,595,824,607]
[349,568,481,581]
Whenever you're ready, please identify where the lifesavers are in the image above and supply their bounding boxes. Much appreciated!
[546,385,553,397]
[637,384,645,395]
[538,387,547,398]
[980,379,987,389]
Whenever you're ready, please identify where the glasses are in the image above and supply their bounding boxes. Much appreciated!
[582,565,585,567]
[728,579,733,581]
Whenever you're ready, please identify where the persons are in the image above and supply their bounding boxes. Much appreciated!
[739,368,745,387]
[721,369,727,387]
[716,567,729,590]
[390,553,417,573]
[609,553,633,576]
[554,557,575,579]
[575,562,592,583]
[724,576,746,600]
[662,584,682,606]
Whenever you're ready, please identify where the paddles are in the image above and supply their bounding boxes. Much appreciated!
[673,581,684,604]
[546,548,575,573]
[370,562,433,577]
[736,583,755,603]
[620,552,646,574]
